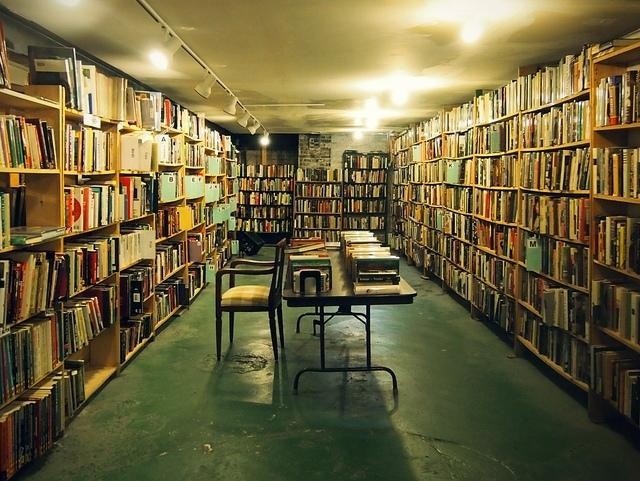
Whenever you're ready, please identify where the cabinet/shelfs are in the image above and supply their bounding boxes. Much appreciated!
[228,151,390,249]
[390,29,639,448]
[0,4,239,481]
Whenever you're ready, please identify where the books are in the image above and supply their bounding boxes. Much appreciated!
[388,30,639,425]
[292,167,343,249]
[341,151,388,243]
[237,163,295,232]
[285,230,400,296]
[0,30,239,480]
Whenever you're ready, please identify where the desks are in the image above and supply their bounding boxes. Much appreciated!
[282,242,418,394]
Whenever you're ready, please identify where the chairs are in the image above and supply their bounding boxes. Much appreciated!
[213,238,287,366]
